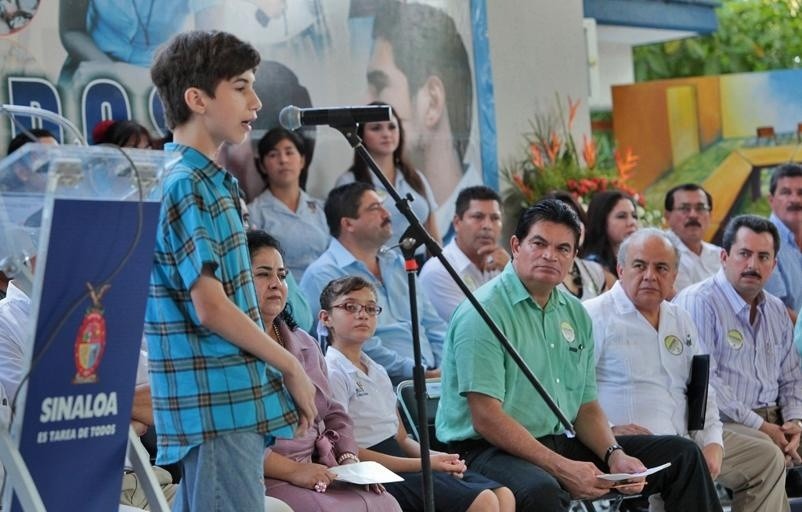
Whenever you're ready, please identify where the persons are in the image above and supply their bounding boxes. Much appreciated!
[0,28,801,512]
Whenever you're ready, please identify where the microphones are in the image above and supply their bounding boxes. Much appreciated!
[276,103,393,132]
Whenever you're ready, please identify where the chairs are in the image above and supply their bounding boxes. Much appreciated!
[396,378,641,511]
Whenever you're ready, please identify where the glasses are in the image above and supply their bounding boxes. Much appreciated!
[326,302,382,316]
[677,204,711,212]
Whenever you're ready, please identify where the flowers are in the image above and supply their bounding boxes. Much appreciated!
[500,95,665,227]
